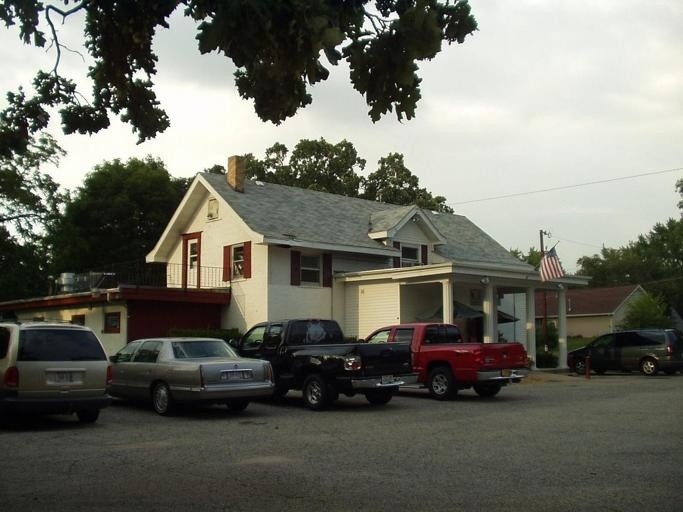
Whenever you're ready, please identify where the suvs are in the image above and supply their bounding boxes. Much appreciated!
[0,319,114,424]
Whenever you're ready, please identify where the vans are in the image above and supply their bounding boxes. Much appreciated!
[566,326,682,377]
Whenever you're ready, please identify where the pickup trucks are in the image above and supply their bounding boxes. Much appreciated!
[227,318,413,412]
[359,321,531,405]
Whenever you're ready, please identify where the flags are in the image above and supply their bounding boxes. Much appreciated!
[538,246,564,283]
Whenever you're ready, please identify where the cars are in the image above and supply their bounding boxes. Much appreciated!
[107,334,275,416]
